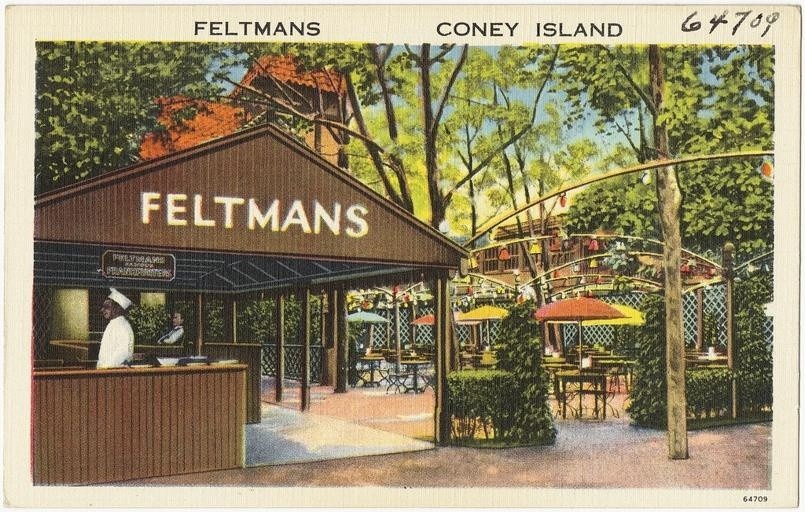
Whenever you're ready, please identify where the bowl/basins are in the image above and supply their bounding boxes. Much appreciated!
[157,358,180,366]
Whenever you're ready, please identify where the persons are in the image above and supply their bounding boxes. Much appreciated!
[156,313,185,346]
[96,288,134,367]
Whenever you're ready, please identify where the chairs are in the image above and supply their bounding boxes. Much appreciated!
[352,343,735,425]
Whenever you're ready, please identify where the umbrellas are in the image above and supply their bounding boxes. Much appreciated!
[346,311,390,325]
[534,296,627,373]
[579,303,647,348]
[410,304,511,347]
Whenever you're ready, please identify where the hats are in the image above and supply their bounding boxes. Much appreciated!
[109,289,133,309]
